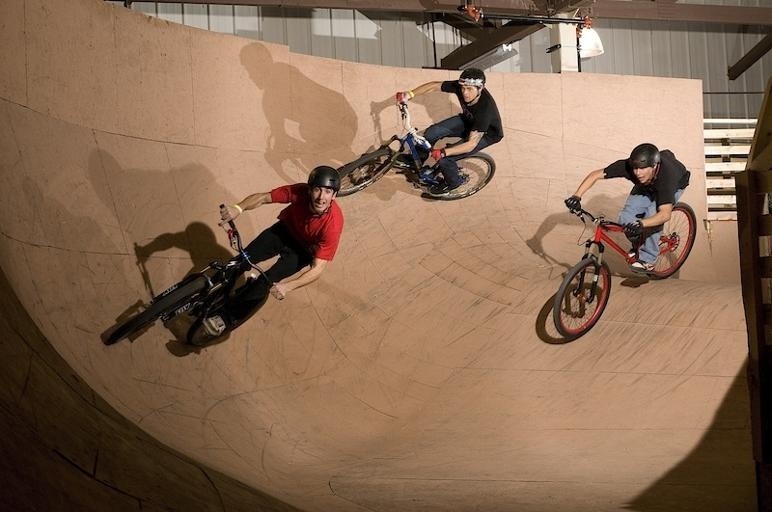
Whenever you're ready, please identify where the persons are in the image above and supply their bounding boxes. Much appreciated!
[565,142,691,274]
[218,165,344,299]
[396,68,504,195]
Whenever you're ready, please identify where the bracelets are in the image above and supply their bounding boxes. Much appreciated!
[409,89,414,98]
[233,203,243,214]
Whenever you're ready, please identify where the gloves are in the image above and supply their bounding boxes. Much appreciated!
[621,220,643,237]
[395,91,412,105]
[564,195,582,212]
[429,148,442,162]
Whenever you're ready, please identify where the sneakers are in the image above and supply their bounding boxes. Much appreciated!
[201,314,228,337]
[630,260,657,275]
[428,178,464,197]
[393,153,425,168]
[627,249,637,259]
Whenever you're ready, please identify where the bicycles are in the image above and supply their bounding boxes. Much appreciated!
[104,201,289,350]
[551,198,699,341]
[334,89,495,201]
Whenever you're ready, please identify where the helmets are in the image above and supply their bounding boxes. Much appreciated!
[629,142,662,170]
[456,67,486,91]
[308,164,341,192]
